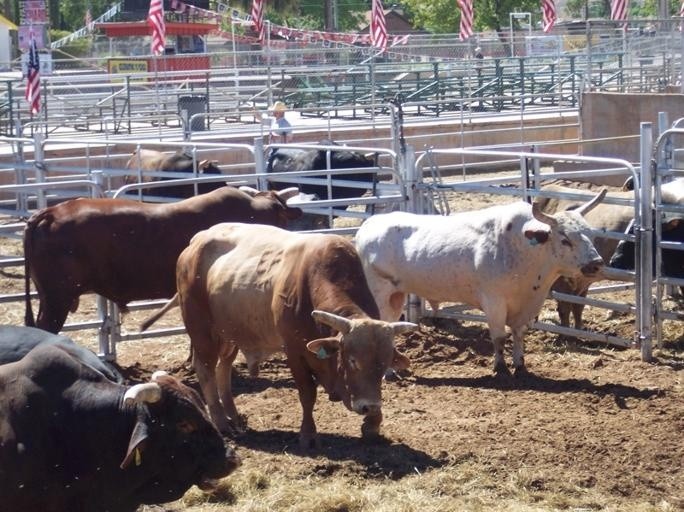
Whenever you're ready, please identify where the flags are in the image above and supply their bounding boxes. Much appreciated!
[455,0,474,44]
[540,0,559,35]
[84,1,93,33]
[678,0,684,33]
[369,0,389,56]
[251,0,266,48]
[146,1,165,61]
[608,0,628,33]
[23,18,41,115]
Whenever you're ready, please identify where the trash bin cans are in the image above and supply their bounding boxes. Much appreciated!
[178,97,206,130]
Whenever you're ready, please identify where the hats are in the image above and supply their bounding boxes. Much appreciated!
[268,101,286,111]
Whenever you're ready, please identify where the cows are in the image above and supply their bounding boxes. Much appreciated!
[0,324,242,512]
[264,142,382,220]
[22,184,303,334]
[139,221,420,455]
[123,148,227,200]
[353,176,684,387]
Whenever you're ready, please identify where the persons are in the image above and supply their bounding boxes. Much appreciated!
[474,47,484,78]
[266,100,291,167]
